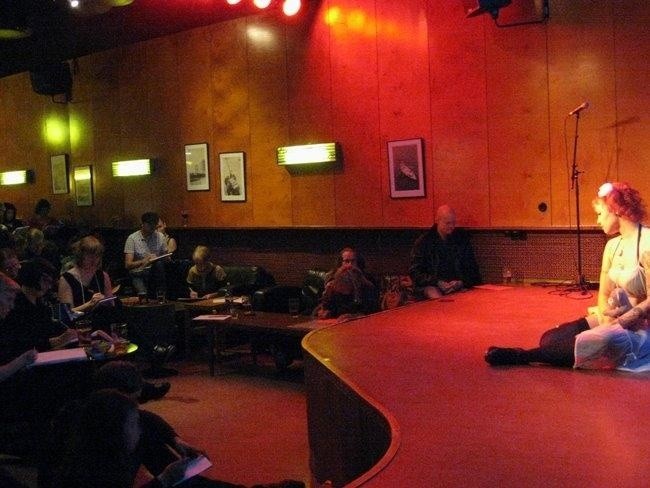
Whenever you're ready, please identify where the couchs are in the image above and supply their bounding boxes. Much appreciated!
[168,258,258,300]
[255,265,382,321]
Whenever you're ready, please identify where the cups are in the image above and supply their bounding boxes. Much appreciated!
[240,294,252,315]
[139,293,149,305]
[155,287,165,304]
[109,322,129,354]
[75,319,94,348]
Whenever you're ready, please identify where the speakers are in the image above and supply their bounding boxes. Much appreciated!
[29,62,73,96]
[463,0,510,18]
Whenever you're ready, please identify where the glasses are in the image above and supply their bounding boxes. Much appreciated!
[341,257,357,264]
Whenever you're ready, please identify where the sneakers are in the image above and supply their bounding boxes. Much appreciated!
[137,382,170,404]
[150,343,177,378]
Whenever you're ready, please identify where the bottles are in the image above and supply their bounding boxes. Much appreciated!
[224,281,234,315]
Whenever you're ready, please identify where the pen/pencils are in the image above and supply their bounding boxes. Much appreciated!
[189,287,194,292]
[209,315,225,317]
[143,252,151,255]
[88,289,95,295]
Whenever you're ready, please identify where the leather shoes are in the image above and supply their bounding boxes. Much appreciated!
[485,346,526,366]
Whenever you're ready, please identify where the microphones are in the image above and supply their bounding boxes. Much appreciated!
[568,101,589,116]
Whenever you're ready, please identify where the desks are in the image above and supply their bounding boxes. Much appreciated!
[108,292,353,378]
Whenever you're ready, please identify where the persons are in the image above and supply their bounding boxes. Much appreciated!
[409,206,482,301]
[484,180,649,373]
[319,265,377,323]
[224,175,240,195]
[286,247,376,371]
[1,199,230,487]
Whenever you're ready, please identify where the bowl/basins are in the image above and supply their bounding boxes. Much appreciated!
[83,339,109,359]
